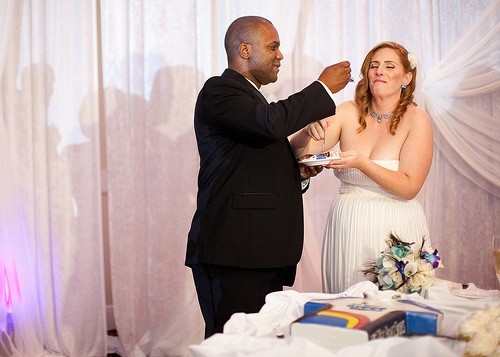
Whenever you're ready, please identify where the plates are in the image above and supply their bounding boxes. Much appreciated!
[298,156,341,166]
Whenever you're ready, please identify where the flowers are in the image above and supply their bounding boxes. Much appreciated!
[362,232,445,300]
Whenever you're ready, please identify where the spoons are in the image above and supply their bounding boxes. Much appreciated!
[350,78,354,82]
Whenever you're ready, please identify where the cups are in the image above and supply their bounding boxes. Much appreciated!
[492,234,500,282]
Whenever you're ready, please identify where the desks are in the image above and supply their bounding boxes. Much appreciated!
[185,276,499,357]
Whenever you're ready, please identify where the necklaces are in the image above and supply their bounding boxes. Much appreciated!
[368,104,395,124]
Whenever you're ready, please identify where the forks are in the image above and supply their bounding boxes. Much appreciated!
[322,129,326,155]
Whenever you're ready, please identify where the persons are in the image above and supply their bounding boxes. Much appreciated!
[184,16,352,339]
[285,41,435,299]
